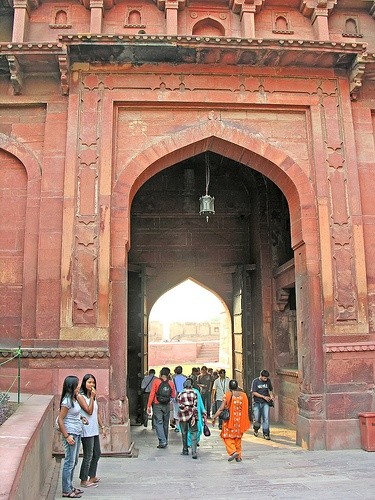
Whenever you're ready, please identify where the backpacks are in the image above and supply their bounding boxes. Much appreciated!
[156,378,172,404]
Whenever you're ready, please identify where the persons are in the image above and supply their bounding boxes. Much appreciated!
[141,366,187,448]
[210,380,250,462]
[76,374,108,488]
[176,378,207,459]
[189,366,231,429]
[251,369,275,440]
[57,376,88,498]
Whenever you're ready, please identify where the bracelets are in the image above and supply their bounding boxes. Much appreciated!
[65,434,69,438]
[102,426,105,428]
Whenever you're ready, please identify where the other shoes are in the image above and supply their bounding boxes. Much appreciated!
[73,488,84,494]
[236,458,242,462]
[228,451,239,462]
[254,429,259,437]
[183,448,188,455]
[192,456,197,459]
[62,490,82,498]
[80,483,98,488]
[157,444,168,448]
[263,433,270,440]
[89,478,100,483]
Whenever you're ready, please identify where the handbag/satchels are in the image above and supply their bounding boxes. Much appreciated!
[269,398,275,408]
[55,415,61,431]
[203,415,211,436]
[219,409,229,420]
